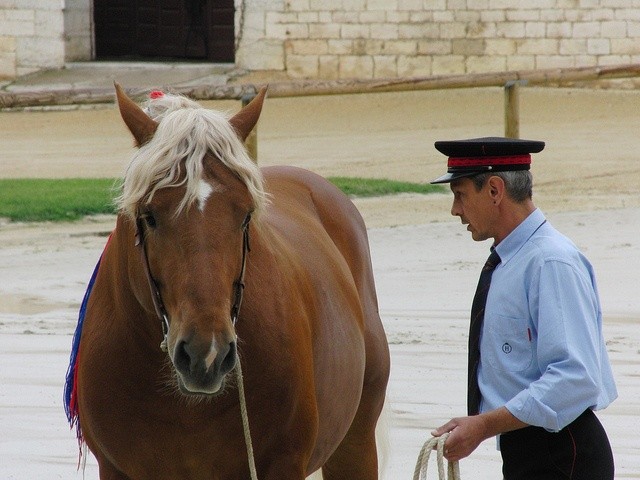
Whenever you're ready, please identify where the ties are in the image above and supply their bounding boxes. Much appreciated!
[467,249,501,416]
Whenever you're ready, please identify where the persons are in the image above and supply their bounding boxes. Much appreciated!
[430,137,618,480]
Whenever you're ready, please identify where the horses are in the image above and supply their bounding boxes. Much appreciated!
[75,80,392,480]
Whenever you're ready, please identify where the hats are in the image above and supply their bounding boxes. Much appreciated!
[430,137,545,184]
